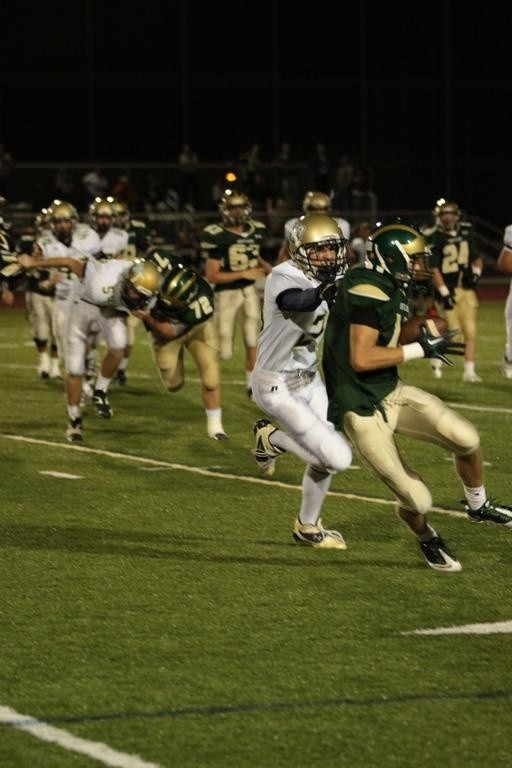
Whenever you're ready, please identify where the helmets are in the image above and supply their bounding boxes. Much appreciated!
[112,203,130,229]
[432,202,462,232]
[161,268,200,305]
[365,224,434,285]
[121,261,165,308]
[35,212,45,226]
[302,191,332,215]
[289,214,348,280]
[219,193,253,224]
[44,201,79,238]
[93,202,113,229]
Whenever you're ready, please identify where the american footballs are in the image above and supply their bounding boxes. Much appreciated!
[399,315,448,347]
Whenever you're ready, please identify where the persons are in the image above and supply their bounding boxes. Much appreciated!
[20,210,64,381]
[252,215,354,548]
[88,202,130,385]
[422,201,483,383]
[133,248,230,441]
[112,204,145,385]
[498,222,511,380]
[77,136,379,215]
[201,194,272,401]
[323,225,512,572]
[19,255,159,445]
[33,203,99,406]
[277,192,351,263]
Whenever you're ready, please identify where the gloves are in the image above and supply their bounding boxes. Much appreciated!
[419,323,466,364]
[443,294,456,309]
[464,271,479,288]
[317,278,337,308]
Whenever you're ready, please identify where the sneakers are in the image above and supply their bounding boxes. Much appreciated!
[91,386,114,419]
[430,356,442,378]
[460,499,512,527]
[416,532,463,572]
[501,357,512,378]
[67,413,83,442]
[49,359,62,379]
[85,349,101,378]
[292,516,347,551]
[247,387,256,404]
[117,369,125,387]
[463,370,481,383]
[207,423,228,441]
[39,355,50,377]
[252,418,287,476]
